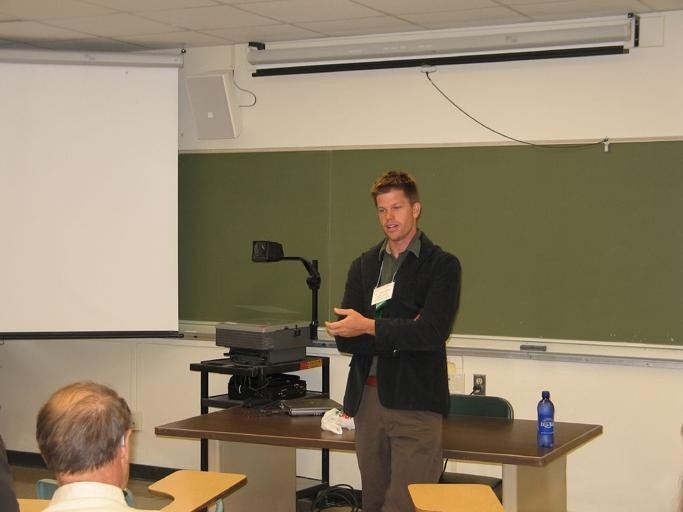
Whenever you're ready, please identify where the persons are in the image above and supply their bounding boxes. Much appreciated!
[325,170,461,512]
[36,383,162,512]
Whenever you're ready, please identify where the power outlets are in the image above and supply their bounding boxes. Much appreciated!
[474,375,485,396]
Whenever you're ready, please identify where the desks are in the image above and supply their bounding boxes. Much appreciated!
[156,404,602,512]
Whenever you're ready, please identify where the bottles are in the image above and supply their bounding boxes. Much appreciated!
[536,390,554,449]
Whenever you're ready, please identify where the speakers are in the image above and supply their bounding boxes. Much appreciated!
[185,68,236,142]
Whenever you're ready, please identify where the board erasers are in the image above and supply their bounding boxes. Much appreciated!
[521,345,547,352]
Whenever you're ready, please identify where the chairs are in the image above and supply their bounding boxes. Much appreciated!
[442,394,514,501]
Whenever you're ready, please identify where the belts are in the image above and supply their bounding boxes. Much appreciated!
[363,376,378,387]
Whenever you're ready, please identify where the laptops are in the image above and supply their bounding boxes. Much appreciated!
[281,398,344,416]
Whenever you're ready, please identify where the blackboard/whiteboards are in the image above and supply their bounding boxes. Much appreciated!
[179,136,683,368]
